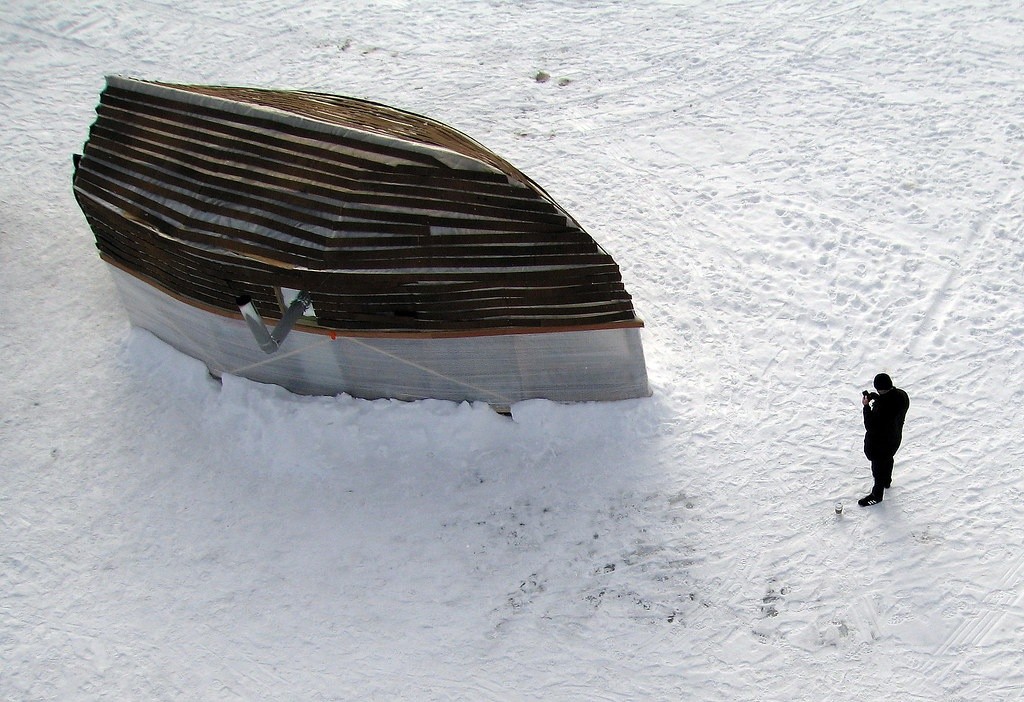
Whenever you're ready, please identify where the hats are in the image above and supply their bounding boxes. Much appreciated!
[874,373,892,390]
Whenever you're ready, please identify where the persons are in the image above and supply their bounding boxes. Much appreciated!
[857,373,910,507]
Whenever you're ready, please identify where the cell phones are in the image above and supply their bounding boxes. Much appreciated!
[862,390,872,402]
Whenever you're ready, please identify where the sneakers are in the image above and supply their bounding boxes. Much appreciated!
[884,484,890,489]
[858,493,883,506]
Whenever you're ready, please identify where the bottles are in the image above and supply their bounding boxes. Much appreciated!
[834,503,843,521]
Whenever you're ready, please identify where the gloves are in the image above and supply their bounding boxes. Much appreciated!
[869,392,879,400]
